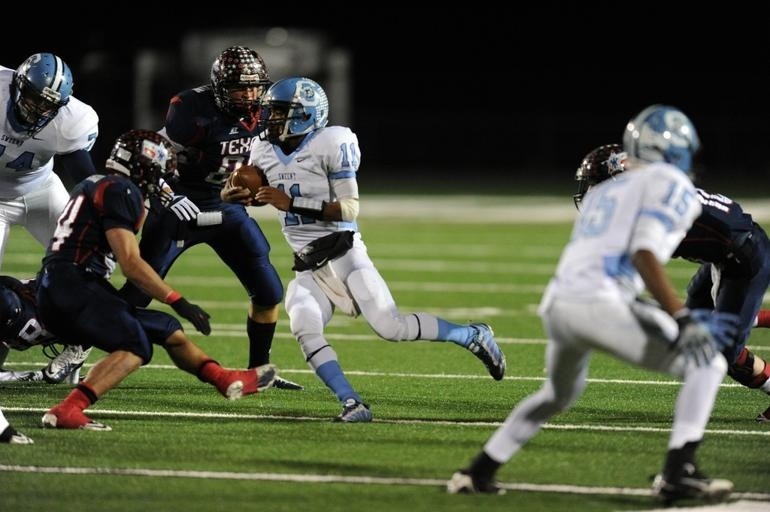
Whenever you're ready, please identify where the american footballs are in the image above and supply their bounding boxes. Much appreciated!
[230,168,270,207]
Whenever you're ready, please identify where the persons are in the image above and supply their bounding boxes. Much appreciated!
[220,78,506,420]
[38,46,303,390]
[572,144,770,422]
[1,275,42,443]
[444,104,734,504]
[1,52,117,384]
[35,129,279,432]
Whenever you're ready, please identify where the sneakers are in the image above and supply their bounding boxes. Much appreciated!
[650,468,736,504]
[273,376,305,393]
[218,363,278,403]
[445,469,508,497]
[467,322,507,382]
[42,342,94,385]
[331,397,373,425]
[41,400,113,433]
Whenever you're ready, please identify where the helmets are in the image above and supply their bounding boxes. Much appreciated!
[8,51,75,138]
[624,102,704,184]
[572,141,629,214]
[106,127,181,216]
[0,288,27,338]
[209,43,270,117]
[258,75,331,145]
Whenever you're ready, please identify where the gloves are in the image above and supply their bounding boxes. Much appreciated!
[171,297,213,336]
[167,193,202,223]
